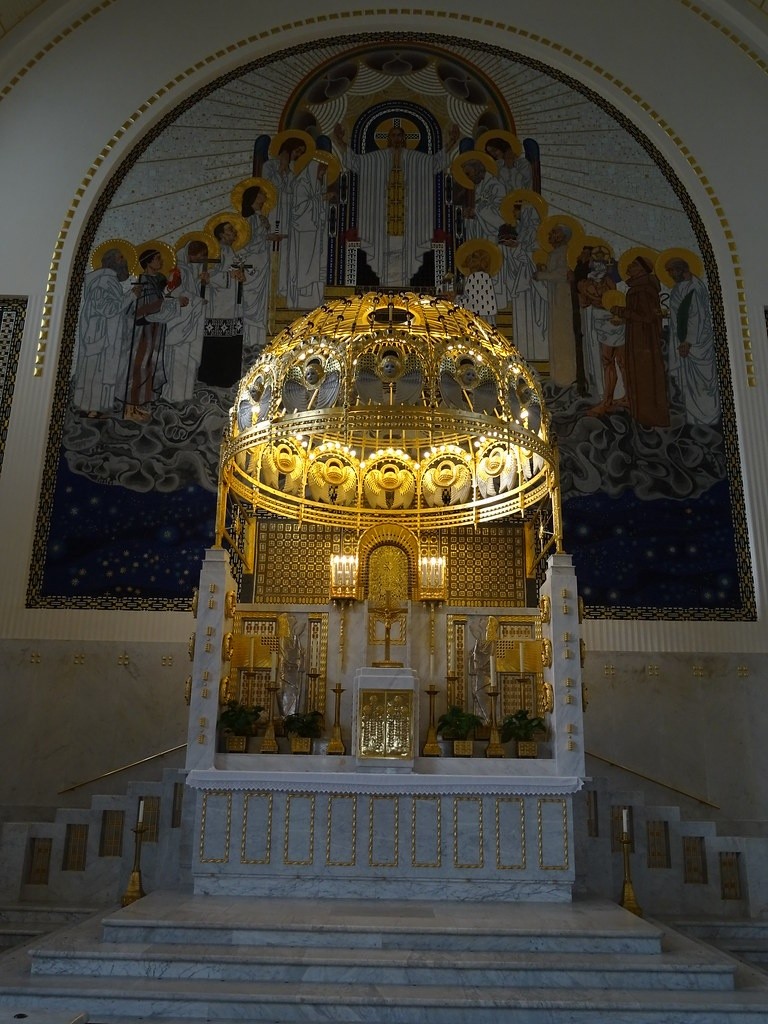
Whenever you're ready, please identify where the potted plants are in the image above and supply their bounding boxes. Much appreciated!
[219,699,265,752]
[281,711,325,752]
[437,704,485,756]
[498,709,547,756]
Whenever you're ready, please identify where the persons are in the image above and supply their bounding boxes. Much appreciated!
[469,616,499,726]
[276,612,306,717]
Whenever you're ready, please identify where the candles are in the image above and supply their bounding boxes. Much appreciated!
[623,810,628,832]
[337,654,341,682]
[312,638,317,669]
[429,655,434,685]
[422,557,444,587]
[520,643,525,673]
[271,654,276,683]
[138,800,144,822]
[490,656,496,686]
[332,555,354,584]
